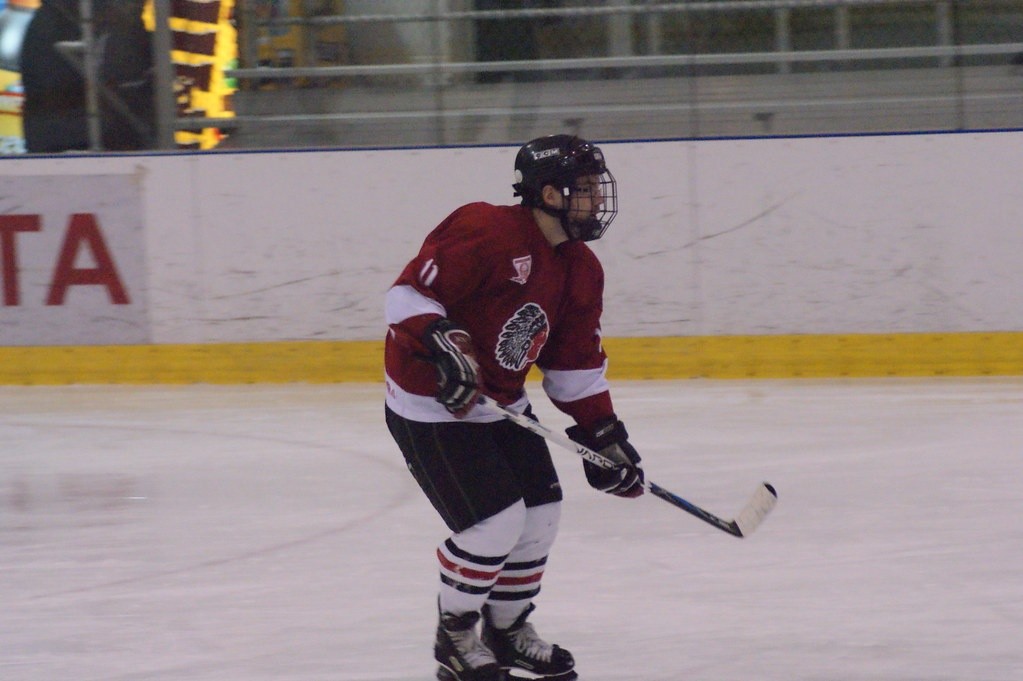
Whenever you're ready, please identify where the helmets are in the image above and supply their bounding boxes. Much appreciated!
[512,134,618,242]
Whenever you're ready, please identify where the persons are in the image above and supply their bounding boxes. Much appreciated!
[383,134,643,681]
[19,0,156,152]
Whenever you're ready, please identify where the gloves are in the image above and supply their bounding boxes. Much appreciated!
[565,414,645,498]
[421,318,483,419]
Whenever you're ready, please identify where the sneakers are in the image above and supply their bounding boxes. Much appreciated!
[434,593,498,681]
[481,600,578,681]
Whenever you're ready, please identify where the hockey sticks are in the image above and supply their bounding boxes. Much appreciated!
[478,392,780,540]
